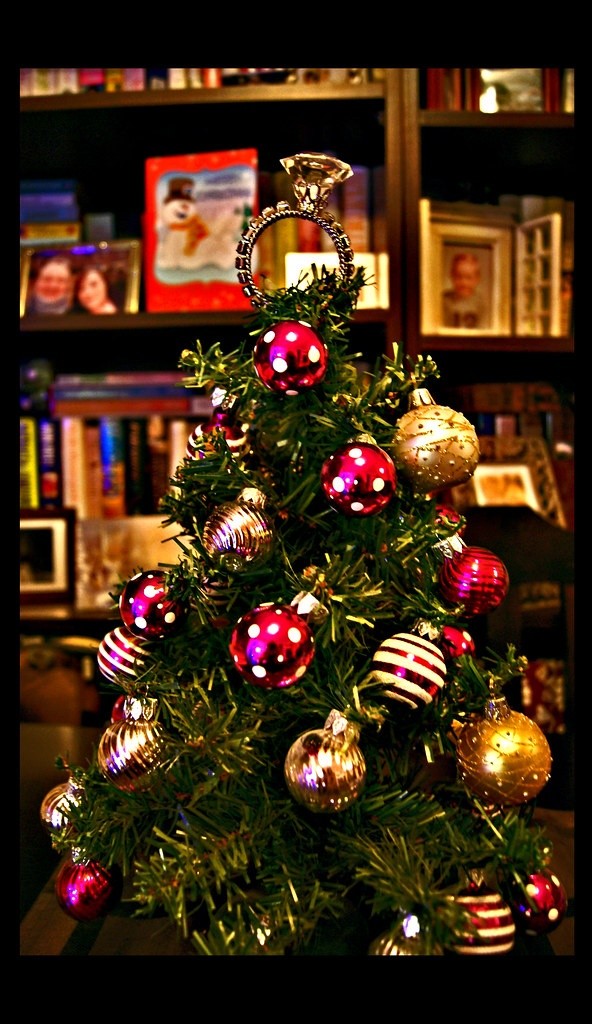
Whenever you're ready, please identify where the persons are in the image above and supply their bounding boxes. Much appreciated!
[25,255,120,315]
[442,250,484,326]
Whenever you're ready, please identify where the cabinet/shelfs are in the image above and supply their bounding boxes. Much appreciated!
[405,69,572,831]
[16,66,406,777]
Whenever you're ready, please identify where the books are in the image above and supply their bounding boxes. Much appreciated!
[257,166,370,291]
[19,369,218,522]
[498,194,572,334]
[19,69,375,94]
[464,413,554,436]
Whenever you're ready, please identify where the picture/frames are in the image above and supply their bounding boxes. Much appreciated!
[18,506,78,609]
[450,434,564,530]
[418,198,511,337]
[463,69,559,111]
[18,238,141,319]
